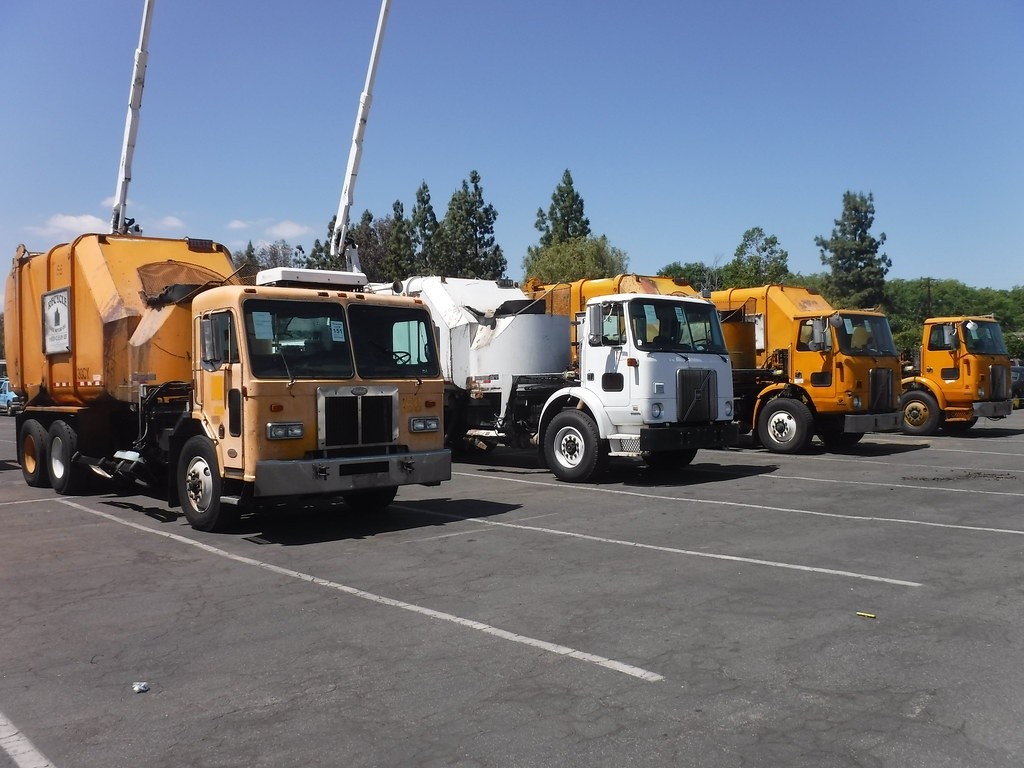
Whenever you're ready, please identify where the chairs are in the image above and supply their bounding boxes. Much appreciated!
[653,336,668,349]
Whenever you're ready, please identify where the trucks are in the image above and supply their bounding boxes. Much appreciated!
[363,275,734,482]
[703,286,1012,436]
[3,231,452,530]
[523,277,905,454]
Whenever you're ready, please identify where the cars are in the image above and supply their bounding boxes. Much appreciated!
[0,377,24,416]
